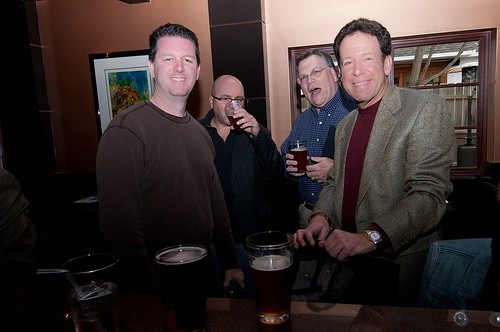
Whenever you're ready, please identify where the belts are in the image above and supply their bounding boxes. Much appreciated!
[304,202,315,211]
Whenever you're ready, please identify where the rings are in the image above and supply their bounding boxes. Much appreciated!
[249,116,252,121]
[314,176,317,181]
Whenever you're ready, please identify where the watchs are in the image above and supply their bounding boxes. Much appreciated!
[362,229,385,251]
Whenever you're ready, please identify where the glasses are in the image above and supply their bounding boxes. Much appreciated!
[212,95,246,103]
[297,66,330,85]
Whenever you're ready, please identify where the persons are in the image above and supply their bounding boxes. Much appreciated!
[95,22,245,300]
[196,75,290,299]
[280,48,359,302]
[462,68,478,81]
[287,19,459,303]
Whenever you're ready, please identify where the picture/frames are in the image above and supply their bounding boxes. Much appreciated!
[87,48,155,144]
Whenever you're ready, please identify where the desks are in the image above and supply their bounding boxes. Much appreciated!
[64,293,500,332]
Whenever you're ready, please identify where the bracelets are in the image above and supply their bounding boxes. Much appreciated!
[310,214,332,227]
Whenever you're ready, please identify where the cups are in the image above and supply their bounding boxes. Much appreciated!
[224,101,248,132]
[153,243,209,332]
[246,231,294,324]
[58,254,121,332]
[288,139,308,177]
[225,287,244,299]
